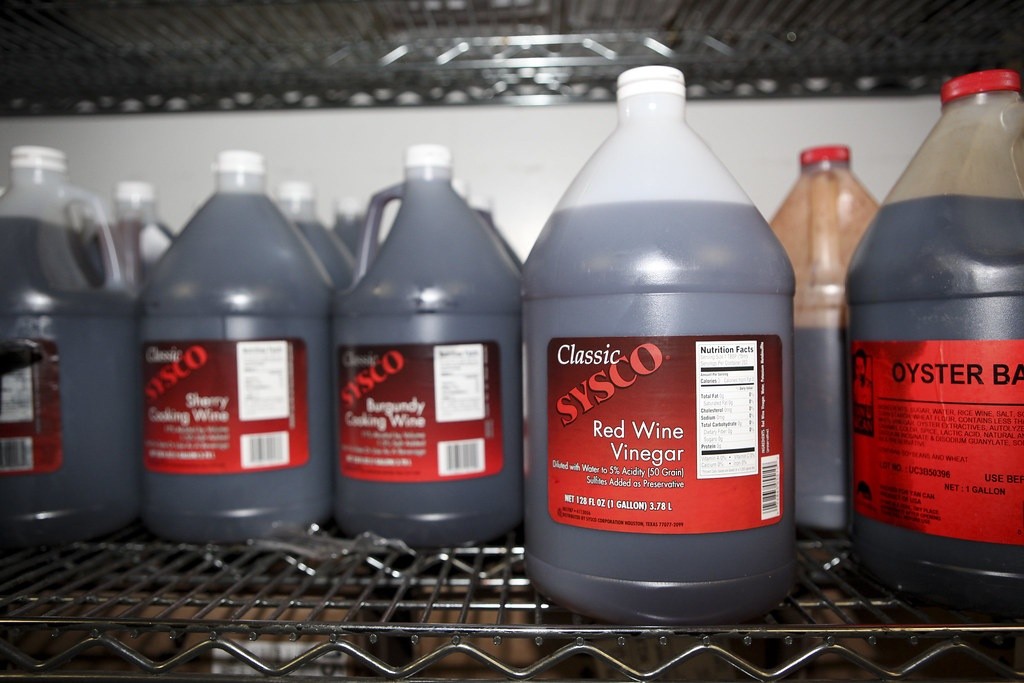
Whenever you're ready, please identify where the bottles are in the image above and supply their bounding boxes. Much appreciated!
[277,181,332,277]
[334,195,370,263]
[332,144,519,548]
[846,68,1024,601]
[520,66,796,623]
[117,180,179,293]
[143,150,336,544]
[0,144,152,545]
[471,199,524,271]
[770,146,879,530]
